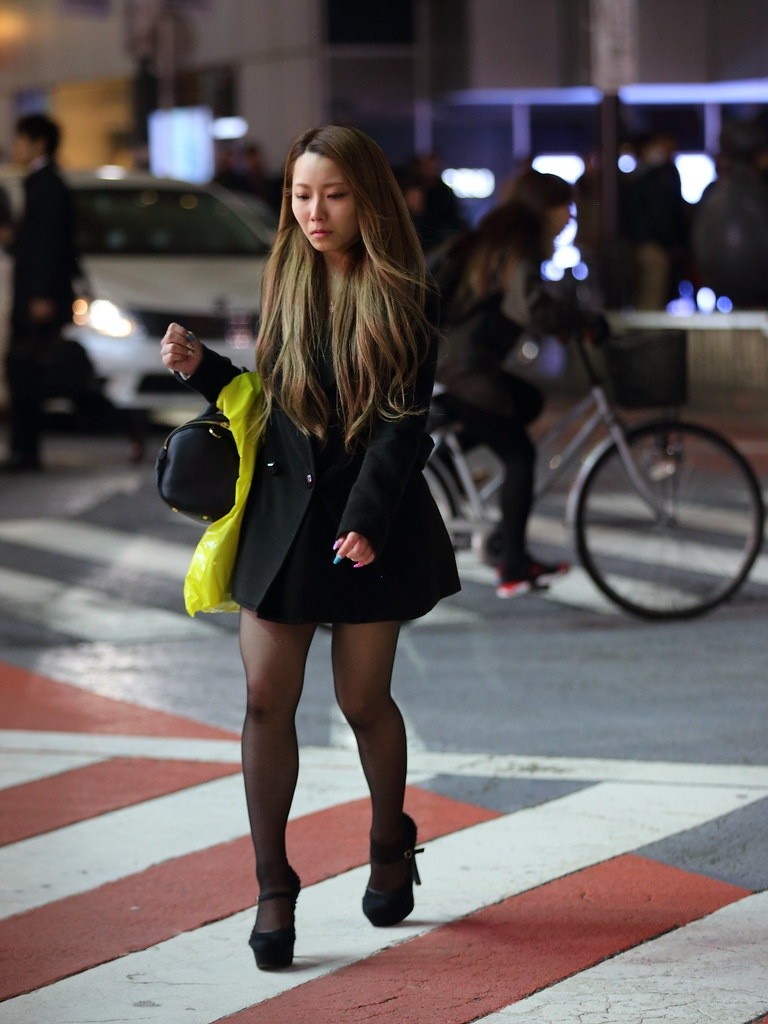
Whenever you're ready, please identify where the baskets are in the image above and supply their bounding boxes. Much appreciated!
[604,327,691,411]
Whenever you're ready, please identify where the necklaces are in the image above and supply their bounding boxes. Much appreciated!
[328,298,335,313]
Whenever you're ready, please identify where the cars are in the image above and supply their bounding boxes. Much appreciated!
[1,159,282,431]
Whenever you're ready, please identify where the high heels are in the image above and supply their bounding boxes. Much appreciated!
[360,814,422,924]
[250,864,303,968]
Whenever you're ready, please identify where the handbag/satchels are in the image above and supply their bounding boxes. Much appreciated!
[155,355,237,523]
[182,369,269,630]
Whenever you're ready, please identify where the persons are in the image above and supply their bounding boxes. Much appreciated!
[0,113,86,476]
[575,125,768,312]
[423,168,611,599]
[161,125,462,973]
[399,155,469,256]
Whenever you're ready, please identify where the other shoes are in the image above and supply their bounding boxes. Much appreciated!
[0,456,45,474]
[124,407,153,460]
[481,526,571,598]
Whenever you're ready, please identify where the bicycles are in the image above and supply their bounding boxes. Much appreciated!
[425,307,764,622]
